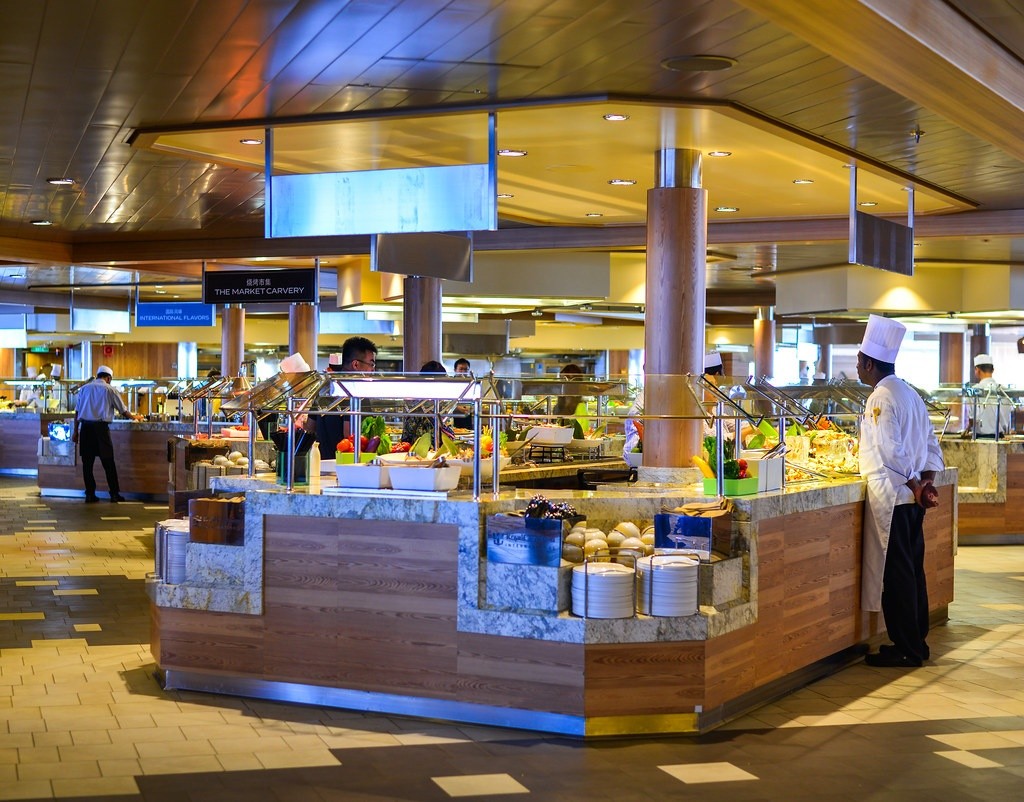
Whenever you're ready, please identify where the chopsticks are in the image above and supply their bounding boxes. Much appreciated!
[271,427,317,456]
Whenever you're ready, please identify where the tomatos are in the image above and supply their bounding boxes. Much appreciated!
[336,435,369,453]
[737,459,748,478]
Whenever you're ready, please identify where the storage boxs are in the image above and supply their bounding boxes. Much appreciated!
[335,462,388,488]
[485,515,586,568]
[653,514,737,564]
[744,457,783,491]
[388,466,462,491]
[702,476,759,495]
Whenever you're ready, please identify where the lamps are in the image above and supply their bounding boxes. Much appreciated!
[337,253,610,314]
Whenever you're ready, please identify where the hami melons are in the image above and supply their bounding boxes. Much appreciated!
[213,451,249,468]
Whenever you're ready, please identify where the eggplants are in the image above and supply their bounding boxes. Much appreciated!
[365,435,380,452]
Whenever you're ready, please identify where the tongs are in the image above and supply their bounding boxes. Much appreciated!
[761,442,786,459]
[366,459,384,466]
[425,456,444,468]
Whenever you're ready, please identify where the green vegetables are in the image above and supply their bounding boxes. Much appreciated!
[361,416,391,454]
[705,435,740,478]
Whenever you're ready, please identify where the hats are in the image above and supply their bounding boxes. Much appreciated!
[973,354,993,367]
[51,364,61,376]
[98,366,113,376]
[705,352,722,368]
[859,313,906,364]
[27,367,37,378]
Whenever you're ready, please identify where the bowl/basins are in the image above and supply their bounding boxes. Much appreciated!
[445,457,511,475]
[388,466,462,490]
[334,463,391,488]
[336,451,377,464]
[522,426,575,447]
[703,456,783,496]
[561,520,586,563]
[584,522,655,567]
[380,452,446,466]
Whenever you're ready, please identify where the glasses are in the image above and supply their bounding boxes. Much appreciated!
[355,359,375,368]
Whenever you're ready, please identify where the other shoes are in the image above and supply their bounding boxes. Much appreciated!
[865,647,923,668]
[878,643,930,661]
[85,495,100,503]
[111,495,125,503]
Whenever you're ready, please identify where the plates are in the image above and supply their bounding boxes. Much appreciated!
[155,518,189,585]
[636,556,699,617]
[565,437,603,448]
[571,563,635,619]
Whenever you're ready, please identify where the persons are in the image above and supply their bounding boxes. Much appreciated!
[12,337,824,504]
[855,311,943,668]
[961,354,1015,439]
